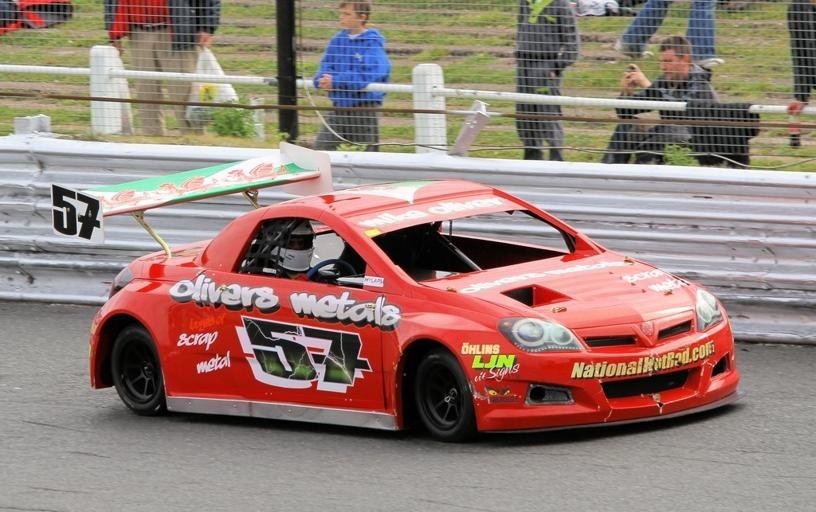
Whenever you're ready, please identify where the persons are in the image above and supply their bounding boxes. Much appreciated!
[786,0,816,103]
[312,1,392,150]
[513,0,579,162]
[616,0,726,69]
[263,219,322,282]
[102,0,224,138]
[601,36,719,164]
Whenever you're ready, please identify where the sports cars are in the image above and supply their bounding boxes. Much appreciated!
[86,179,740,441]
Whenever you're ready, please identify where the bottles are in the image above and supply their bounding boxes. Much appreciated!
[788,110,802,148]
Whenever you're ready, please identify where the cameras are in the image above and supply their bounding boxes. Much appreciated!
[624,66,635,79]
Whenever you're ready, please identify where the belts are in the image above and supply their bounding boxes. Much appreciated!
[130,22,170,32]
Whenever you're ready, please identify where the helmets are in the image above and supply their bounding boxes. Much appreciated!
[268,220,315,272]
